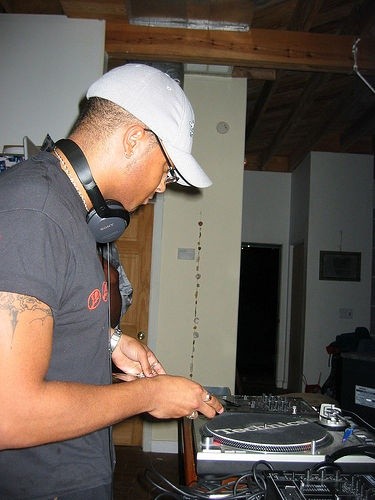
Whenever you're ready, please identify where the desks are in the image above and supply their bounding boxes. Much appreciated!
[340,351,375,428]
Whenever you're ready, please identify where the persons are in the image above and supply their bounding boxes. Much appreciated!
[0,62,224,499]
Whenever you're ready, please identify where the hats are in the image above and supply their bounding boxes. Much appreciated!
[85,62,213,189]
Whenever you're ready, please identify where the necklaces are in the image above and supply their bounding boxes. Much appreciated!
[52,148,90,215]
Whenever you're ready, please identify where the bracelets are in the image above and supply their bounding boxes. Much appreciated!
[109,328,122,356]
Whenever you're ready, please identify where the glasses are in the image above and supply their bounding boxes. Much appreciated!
[144,128,180,185]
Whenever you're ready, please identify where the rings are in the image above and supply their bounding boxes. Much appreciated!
[151,361,159,368]
[203,392,212,402]
[136,372,145,378]
[187,412,198,420]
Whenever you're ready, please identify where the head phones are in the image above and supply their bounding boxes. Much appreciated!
[56,138,130,244]
